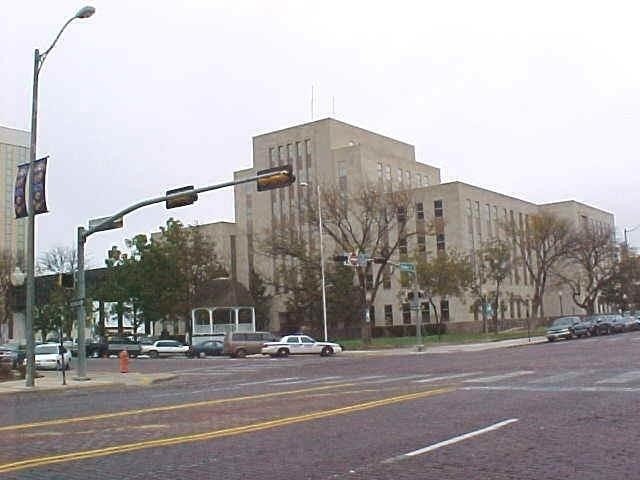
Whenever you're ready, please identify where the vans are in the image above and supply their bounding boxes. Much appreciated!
[224,331,280,358]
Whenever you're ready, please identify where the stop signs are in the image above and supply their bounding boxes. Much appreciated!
[349,253,359,265]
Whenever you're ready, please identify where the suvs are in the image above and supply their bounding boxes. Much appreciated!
[546,316,581,343]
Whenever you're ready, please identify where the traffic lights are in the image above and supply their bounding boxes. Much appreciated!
[330,255,348,262]
[373,257,387,264]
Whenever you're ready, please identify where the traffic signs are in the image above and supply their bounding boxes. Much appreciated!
[68,298,85,308]
[399,263,416,272]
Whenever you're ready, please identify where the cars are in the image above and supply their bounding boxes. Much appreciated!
[573,313,640,338]
[260,334,343,357]
[1,339,224,371]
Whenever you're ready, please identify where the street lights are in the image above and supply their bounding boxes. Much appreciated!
[299,181,328,341]
[22,4,97,389]
[558,289,563,317]
[9,265,25,306]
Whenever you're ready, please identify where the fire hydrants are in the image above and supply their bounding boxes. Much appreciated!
[117,349,130,373]
